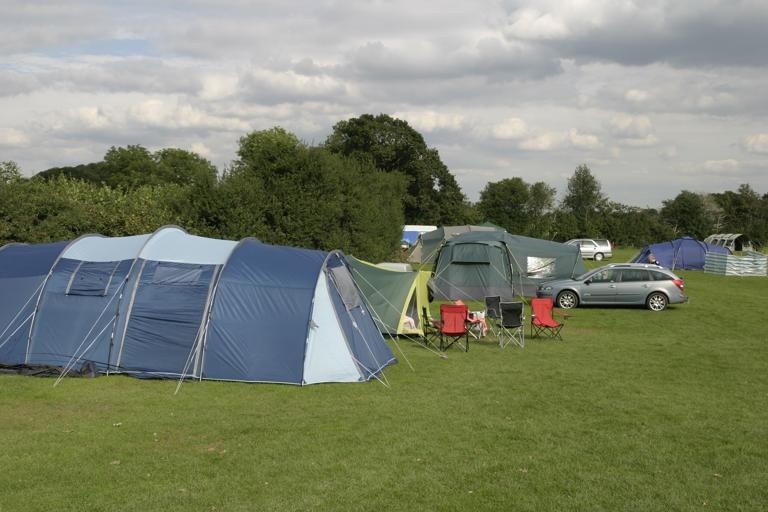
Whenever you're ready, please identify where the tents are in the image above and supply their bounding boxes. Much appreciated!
[629,235,730,271]
[405,222,508,265]
[1,221,397,387]
[345,254,439,339]
[430,230,588,302]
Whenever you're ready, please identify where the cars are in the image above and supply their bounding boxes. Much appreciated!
[536,263,688,312]
[565,238,612,261]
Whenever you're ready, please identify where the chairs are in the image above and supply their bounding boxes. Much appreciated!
[421,296,565,353]
